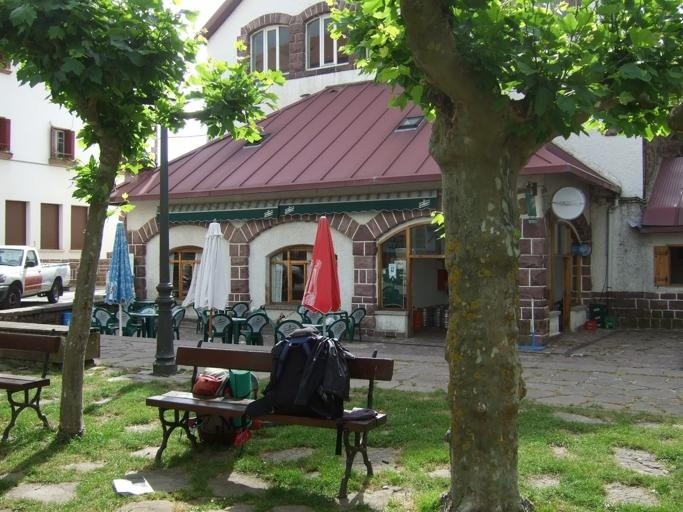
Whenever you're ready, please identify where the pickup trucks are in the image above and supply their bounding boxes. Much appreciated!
[0,245,71,308]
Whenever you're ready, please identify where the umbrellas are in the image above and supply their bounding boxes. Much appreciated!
[300,215,341,338]
[179,217,227,343]
[103,217,138,337]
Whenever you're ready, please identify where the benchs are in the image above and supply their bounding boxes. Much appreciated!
[145,340,394,498]
[0,327,62,441]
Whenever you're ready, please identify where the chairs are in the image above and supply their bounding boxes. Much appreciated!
[90,296,367,345]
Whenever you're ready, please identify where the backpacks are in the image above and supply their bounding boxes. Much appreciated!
[263,330,350,418]
[192,371,261,447]
